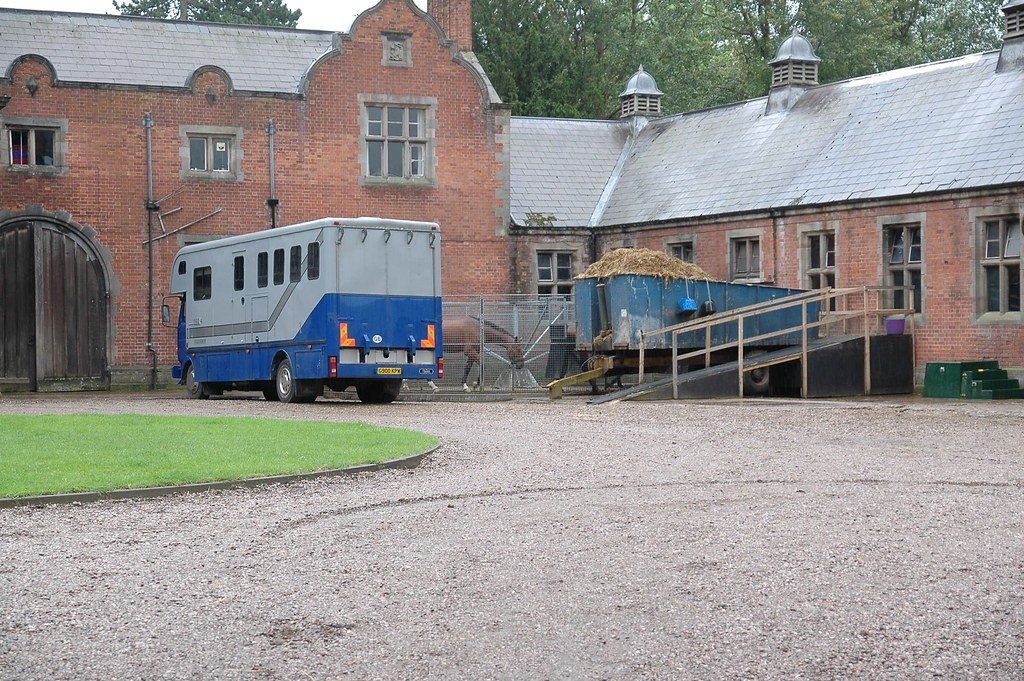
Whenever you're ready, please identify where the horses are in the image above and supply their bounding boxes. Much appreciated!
[402,315,524,394]
[556,331,623,395]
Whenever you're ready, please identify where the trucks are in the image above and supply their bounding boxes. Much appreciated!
[160,217,444,403]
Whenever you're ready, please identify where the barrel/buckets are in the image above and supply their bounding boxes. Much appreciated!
[884,318,905,334]
